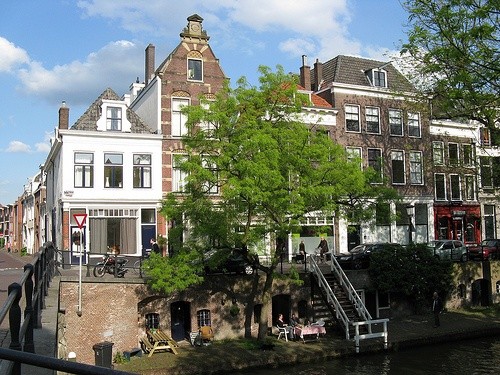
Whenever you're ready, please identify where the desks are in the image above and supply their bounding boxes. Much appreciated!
[296,326,326,339]
[147,330,178,358]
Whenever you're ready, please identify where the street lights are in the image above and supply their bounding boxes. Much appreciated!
[406,203,417,263]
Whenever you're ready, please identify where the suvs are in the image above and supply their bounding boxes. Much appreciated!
[333,242,407,269]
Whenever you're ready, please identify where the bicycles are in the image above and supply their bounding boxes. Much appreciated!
[133,256,152,275]
[311,250,334,266]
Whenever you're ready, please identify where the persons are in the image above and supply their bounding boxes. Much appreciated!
[431,292,442,328]
[298,240,307,263]
[149,237,160,255]
[315,236,329,262]
[199,324,214,342]
[291,313,300,327]
[7,241,11,254]
[275,313,294,340]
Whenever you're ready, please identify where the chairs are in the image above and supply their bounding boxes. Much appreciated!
[277,325,291,342]
[198,326,216,346]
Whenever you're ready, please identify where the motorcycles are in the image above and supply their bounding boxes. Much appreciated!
[93,245,129,278]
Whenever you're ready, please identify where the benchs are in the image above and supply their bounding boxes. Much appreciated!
[169,336,181,349]
[140,337,154,351]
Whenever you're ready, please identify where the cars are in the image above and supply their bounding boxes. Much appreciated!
[412,240,467,263]
[192,248,260,275]
[470,239,500,261]
